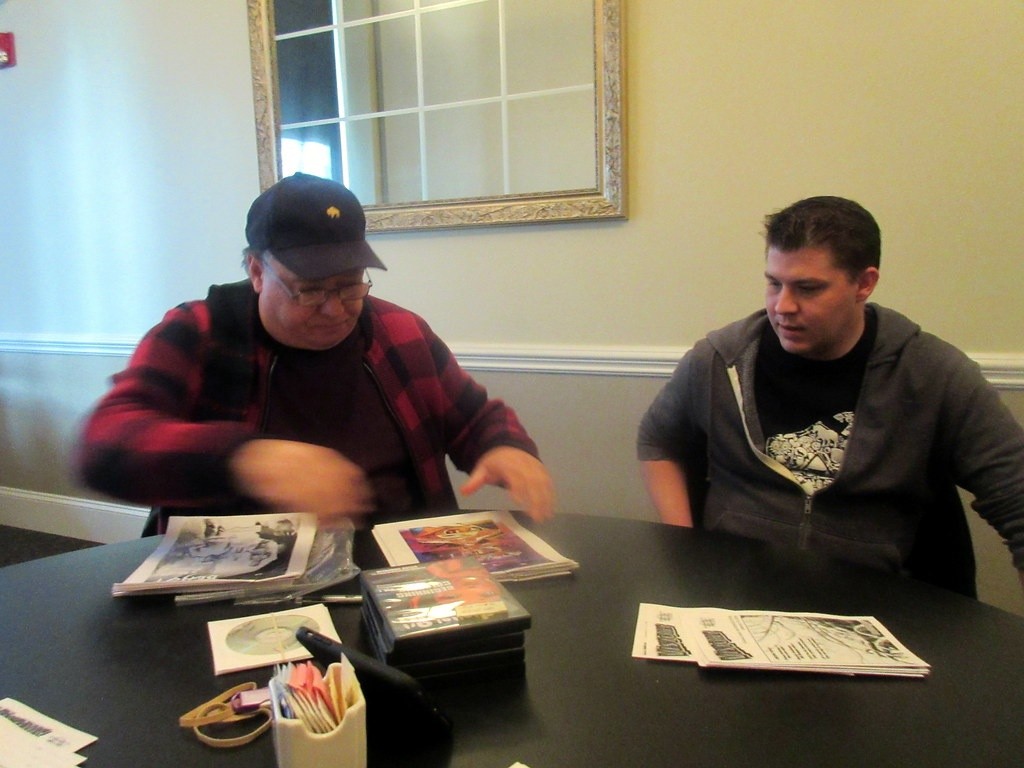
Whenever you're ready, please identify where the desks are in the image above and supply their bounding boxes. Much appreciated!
[1,508,1024,768]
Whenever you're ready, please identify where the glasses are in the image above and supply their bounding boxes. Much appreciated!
[260,255,374,304]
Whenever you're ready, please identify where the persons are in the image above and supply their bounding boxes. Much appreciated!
[143,531,297,582]
[638,197,1024,601]
[78,172,554,524]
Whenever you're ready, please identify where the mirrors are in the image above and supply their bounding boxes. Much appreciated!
[245,0,629,236]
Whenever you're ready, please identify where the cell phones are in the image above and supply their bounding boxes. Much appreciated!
[296,626,453,738]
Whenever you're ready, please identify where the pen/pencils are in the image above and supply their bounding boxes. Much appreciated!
[295,594,363,604]
[173,581,315,606]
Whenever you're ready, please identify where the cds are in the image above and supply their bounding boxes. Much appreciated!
[225,615,319,656]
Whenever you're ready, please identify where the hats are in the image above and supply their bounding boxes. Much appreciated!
[247,172,388,278]
